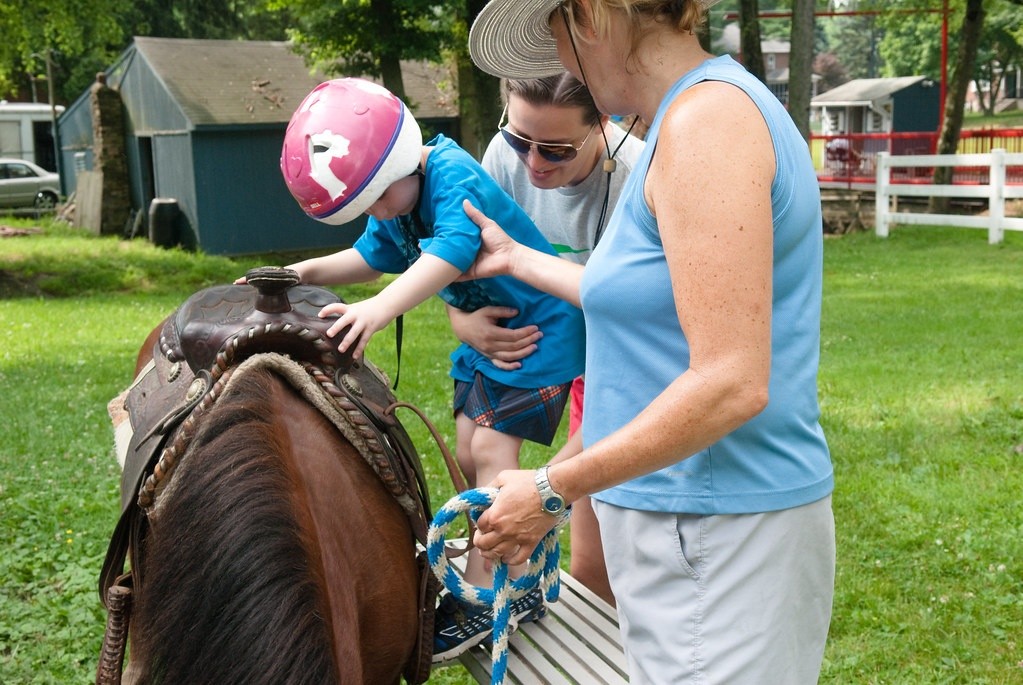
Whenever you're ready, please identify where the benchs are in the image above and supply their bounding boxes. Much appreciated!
[431,534,631,685]
[817,188,897,236]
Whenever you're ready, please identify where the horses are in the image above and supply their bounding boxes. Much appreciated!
[112,312,431,685]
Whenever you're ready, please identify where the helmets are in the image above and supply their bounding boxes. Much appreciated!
[279,77,422,226]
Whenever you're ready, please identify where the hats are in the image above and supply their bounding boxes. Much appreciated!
[466,0,721,80]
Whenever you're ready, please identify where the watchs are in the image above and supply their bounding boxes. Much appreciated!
[535,463,573,516]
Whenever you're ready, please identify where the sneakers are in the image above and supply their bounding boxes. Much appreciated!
[430,593,519,665]
[510,582,548,623]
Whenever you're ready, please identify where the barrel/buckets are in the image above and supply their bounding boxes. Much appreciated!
[149,197,179,250]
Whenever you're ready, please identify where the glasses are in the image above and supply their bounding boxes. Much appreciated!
[498,101,599,164]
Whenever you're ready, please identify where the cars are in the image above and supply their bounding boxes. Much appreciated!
[0,158,62,210]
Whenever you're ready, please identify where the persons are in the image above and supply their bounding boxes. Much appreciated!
[827,128,861,170]
[233,77,586,663]
[465,0,837,685]
[446,67,647,607]
